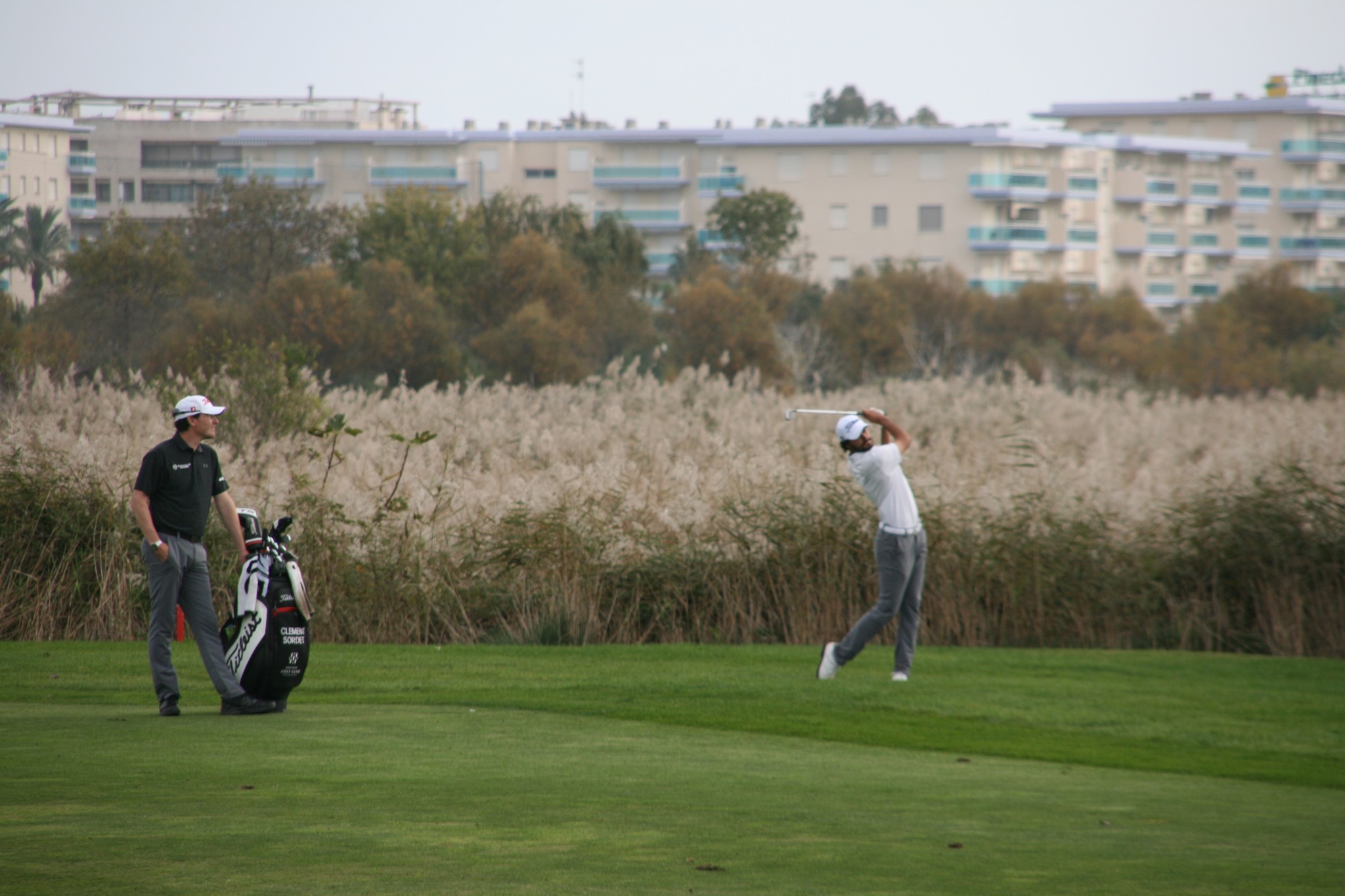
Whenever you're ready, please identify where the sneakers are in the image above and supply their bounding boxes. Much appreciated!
[160,703,181,716]
[221,693,276,714]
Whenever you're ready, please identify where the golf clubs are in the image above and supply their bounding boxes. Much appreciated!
[236,506,298,575]
[784,407,886,421]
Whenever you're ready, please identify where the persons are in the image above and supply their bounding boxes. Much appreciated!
[130,395,279,715]
[814,409,928,683]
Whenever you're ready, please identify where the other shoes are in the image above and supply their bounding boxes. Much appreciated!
[817,642,838,679]
[892,671,907,681]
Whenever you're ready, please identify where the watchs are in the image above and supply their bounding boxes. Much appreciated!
[150,540,162,551]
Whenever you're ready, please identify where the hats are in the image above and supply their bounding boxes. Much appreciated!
[837,414,868,441]
[174,395,225,422]
